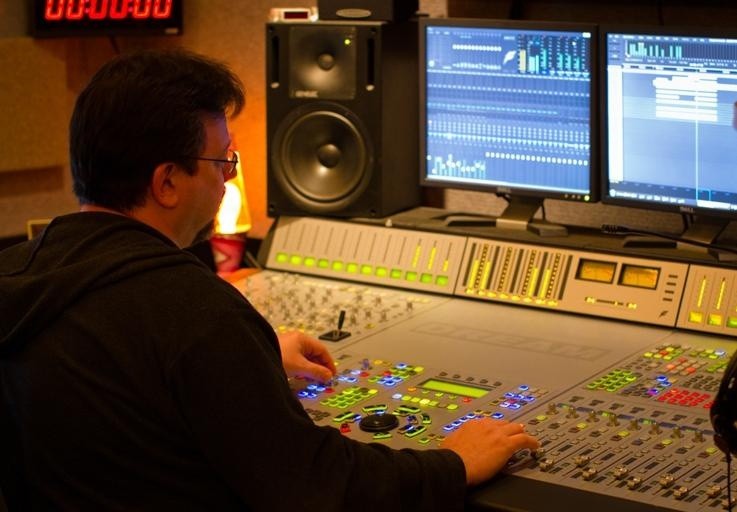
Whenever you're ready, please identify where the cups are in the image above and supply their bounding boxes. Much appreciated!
[210,234,248,273]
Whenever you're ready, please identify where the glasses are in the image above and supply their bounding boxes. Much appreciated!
[194,151,238,174]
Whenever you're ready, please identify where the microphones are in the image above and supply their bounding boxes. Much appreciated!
[600,223,735,254]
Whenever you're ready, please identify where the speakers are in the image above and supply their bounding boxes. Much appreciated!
[266,21,417,218]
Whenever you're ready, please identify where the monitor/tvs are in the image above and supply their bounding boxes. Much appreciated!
[600,26,737,263]
[417,17,599,238]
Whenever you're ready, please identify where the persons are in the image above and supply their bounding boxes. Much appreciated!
[1,46,544,511]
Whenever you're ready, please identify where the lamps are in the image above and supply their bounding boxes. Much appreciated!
[209,150,250,271]
